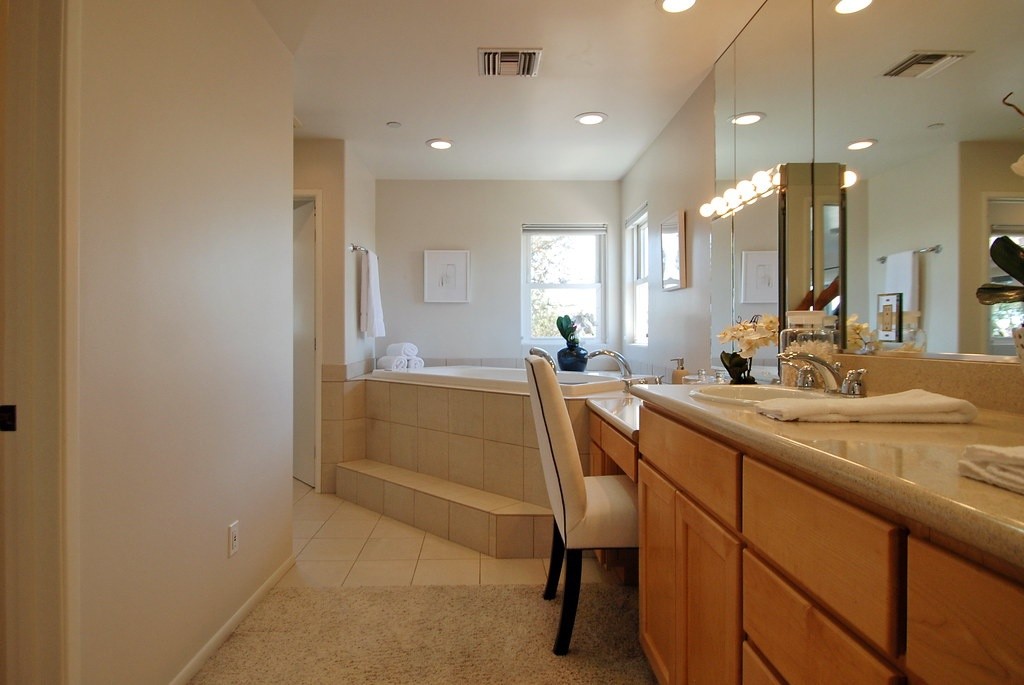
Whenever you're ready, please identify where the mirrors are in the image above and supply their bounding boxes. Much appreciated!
[714,0,1024,384]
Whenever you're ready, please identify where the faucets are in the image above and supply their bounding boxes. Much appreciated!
[527,346,557,384]
[585,349,633,394]
[778,351,868,397]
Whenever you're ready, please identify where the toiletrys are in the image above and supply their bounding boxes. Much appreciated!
[668,357,690,384]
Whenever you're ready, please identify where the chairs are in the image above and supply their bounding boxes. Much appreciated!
[525,355,639,656]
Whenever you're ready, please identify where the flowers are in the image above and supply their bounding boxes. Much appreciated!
[716,314,779,359]
[846,314,882,356]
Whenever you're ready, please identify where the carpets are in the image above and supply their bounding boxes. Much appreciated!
[187,584,659,685]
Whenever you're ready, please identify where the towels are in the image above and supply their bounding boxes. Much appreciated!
[386,343,419,357]
[754,388,980,425]
[958,439,1024,497]
[883,250,921,344]
[375,357,425,370]
[358,247,388,338]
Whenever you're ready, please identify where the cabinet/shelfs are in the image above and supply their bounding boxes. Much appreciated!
[638,407,743,684]
[743,456,905,685]
[588,409,638,572]
[905,536,1024,685]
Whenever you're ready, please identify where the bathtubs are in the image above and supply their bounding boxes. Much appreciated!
[336,356,660,513]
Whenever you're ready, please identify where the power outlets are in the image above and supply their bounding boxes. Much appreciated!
[876,293,903,343]
[228,520,238,556]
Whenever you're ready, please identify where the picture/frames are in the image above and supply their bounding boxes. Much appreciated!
[659,209,686,291]
[424,250,470,303]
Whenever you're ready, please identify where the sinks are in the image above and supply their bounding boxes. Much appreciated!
[686,385,849,407]
